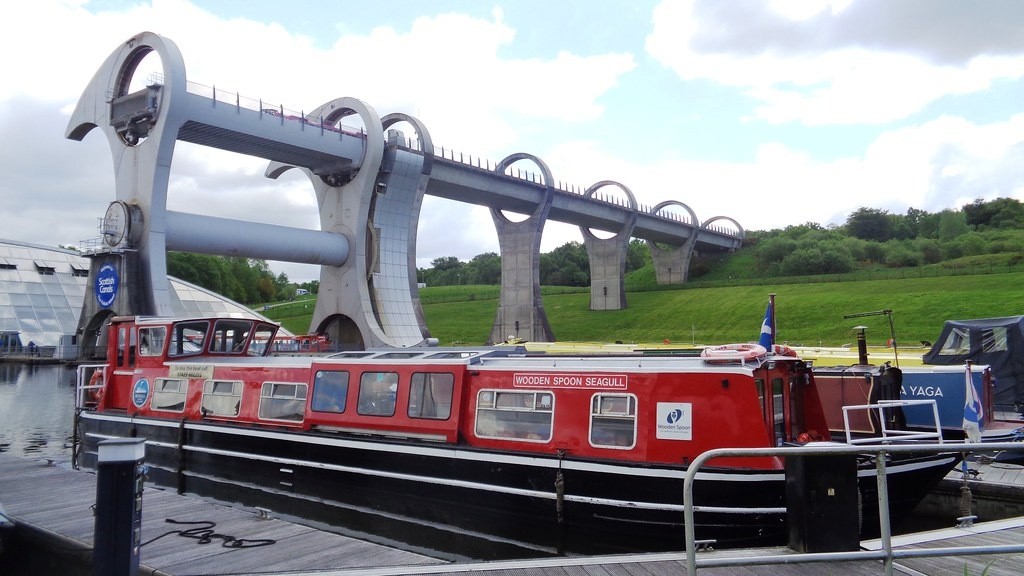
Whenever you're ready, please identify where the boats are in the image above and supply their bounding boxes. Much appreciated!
[72,291,1024,539]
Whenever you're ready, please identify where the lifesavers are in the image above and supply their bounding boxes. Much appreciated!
[771,345,795,356]
[701,343,767,364]
[89,370,103,402]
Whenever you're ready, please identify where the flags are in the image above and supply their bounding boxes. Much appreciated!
[963,366,986,443]
[759,300,772,352]
